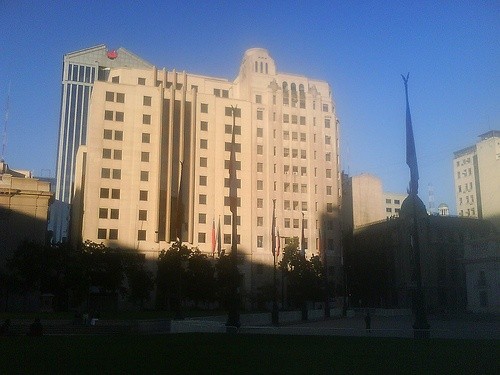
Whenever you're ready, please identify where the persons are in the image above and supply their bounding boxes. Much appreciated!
[74,311,83,320]
[34,318,43,335]
[364,311,371,333]
[90,316,99,328]
